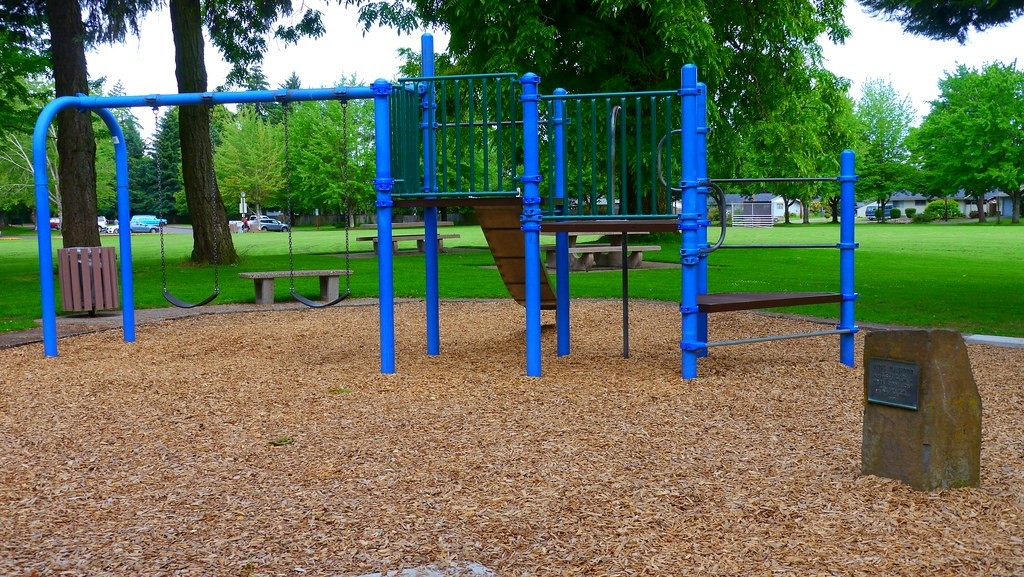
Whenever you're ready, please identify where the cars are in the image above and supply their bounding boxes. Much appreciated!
[130,222,159,234]
[50,217,60,230]
[98,216,120,234]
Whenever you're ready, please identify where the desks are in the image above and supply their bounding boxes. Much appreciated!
[543,231,650,270]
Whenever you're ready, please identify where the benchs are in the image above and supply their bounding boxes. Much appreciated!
[239,267,354,301]
[540,241,662,269]
[358,231,461,252]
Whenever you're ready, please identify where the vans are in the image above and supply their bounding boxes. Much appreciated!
[865,204,893,220]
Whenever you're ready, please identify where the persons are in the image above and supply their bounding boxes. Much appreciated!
[241,217,250,232]
[114,218,119,225]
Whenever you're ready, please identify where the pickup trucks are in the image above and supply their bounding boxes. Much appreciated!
[228,215,270,233]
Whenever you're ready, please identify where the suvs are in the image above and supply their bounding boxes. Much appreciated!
[250,218,289,233]
[131,215,168,227]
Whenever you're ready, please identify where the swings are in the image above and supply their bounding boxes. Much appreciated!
[282,101,352,308]
[153,107,220,308]
